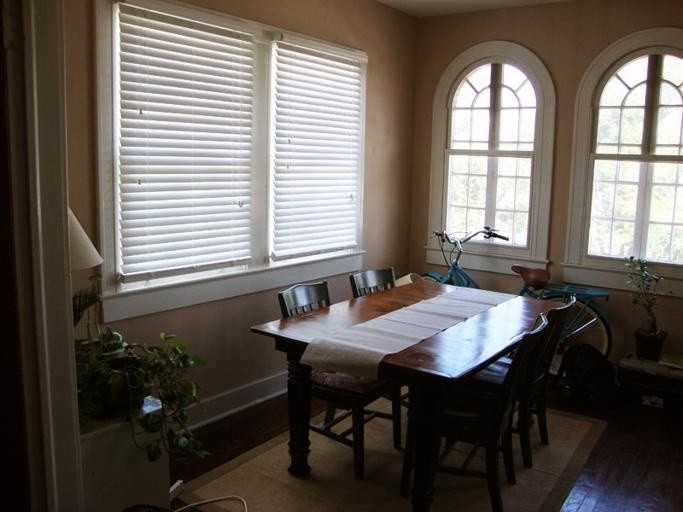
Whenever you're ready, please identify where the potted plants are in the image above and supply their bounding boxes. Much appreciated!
[73,283,213,466]
[620,252,673,362]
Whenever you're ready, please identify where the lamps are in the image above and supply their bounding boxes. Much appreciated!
[66,202,106,275]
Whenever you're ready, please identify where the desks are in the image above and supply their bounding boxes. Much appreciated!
[613,351,680,419]
[248,280,565,512]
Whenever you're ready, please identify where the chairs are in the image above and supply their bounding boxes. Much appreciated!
[274,278,402,480]
[485,293,575,470]
[346,263,411,411]
[398,311,550,512]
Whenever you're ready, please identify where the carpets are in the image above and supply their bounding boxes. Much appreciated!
[179,382,611,512]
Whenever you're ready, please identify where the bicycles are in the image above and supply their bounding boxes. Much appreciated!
[420,224,612,389]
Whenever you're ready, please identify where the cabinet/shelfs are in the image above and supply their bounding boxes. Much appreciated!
[77,393,172,512]
